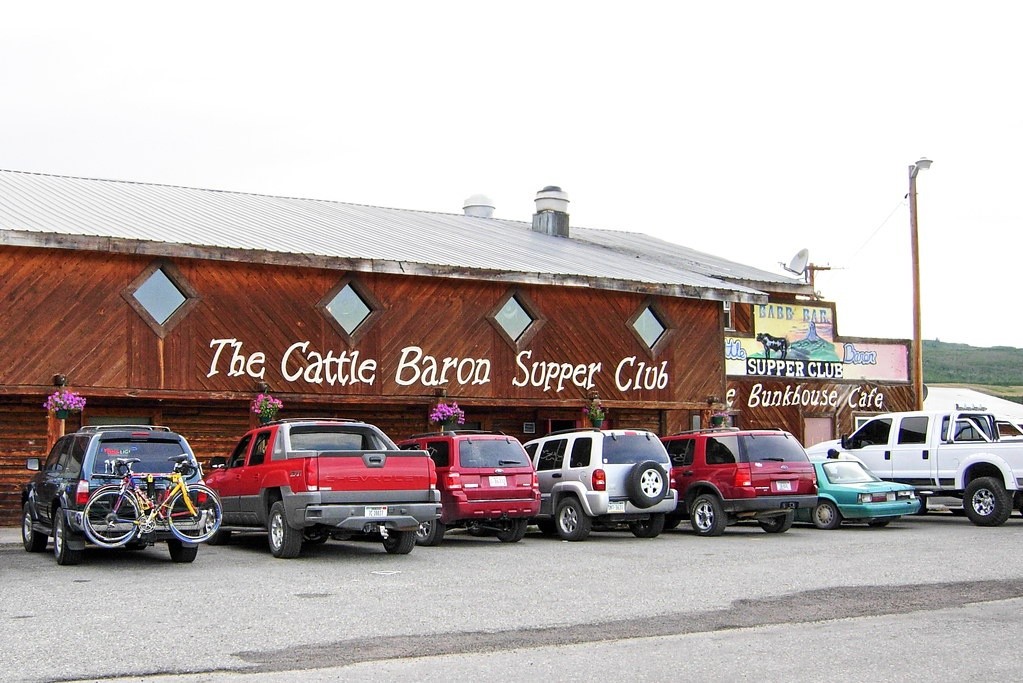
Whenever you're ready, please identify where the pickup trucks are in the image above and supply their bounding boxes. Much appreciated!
[203,417,443,559]
[804,404,1023,527]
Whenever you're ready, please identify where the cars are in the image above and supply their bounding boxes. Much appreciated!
[794,448,924,530]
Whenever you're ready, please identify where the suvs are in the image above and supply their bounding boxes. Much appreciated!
[20,425,208,566]
[658,427,818,537]
[521,427,679,542]
[393,429,542,548]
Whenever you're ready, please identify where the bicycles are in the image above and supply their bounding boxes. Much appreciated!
[82,454,224,549]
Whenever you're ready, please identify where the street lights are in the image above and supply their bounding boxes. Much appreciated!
[908,155,934,411]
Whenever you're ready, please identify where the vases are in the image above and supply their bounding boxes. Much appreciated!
[55,410,69,418]
[710,416,724,424]
[258,416,272,422]
[591,419,602,428]
[439,418,454,425]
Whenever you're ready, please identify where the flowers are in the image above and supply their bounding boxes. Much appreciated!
[583,402,606,420]
[43,390,87,414]
[429,400,466,425]
[251,395,283,418]
[710,412,729,431]
